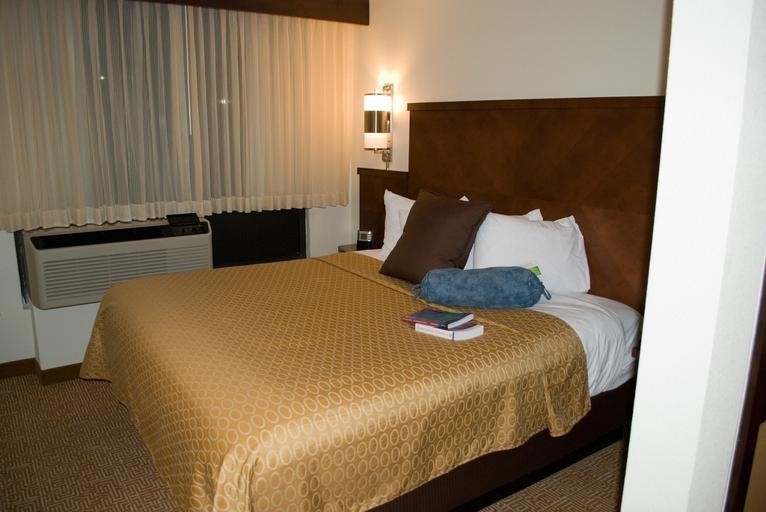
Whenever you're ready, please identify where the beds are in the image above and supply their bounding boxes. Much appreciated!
[83,97,665,512]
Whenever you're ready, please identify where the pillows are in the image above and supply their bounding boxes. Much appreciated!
[376,188,591,314]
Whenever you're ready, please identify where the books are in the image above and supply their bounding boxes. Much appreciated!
[400,308,484,340]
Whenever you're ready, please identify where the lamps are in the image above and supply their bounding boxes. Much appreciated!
[362,81,394,169]
